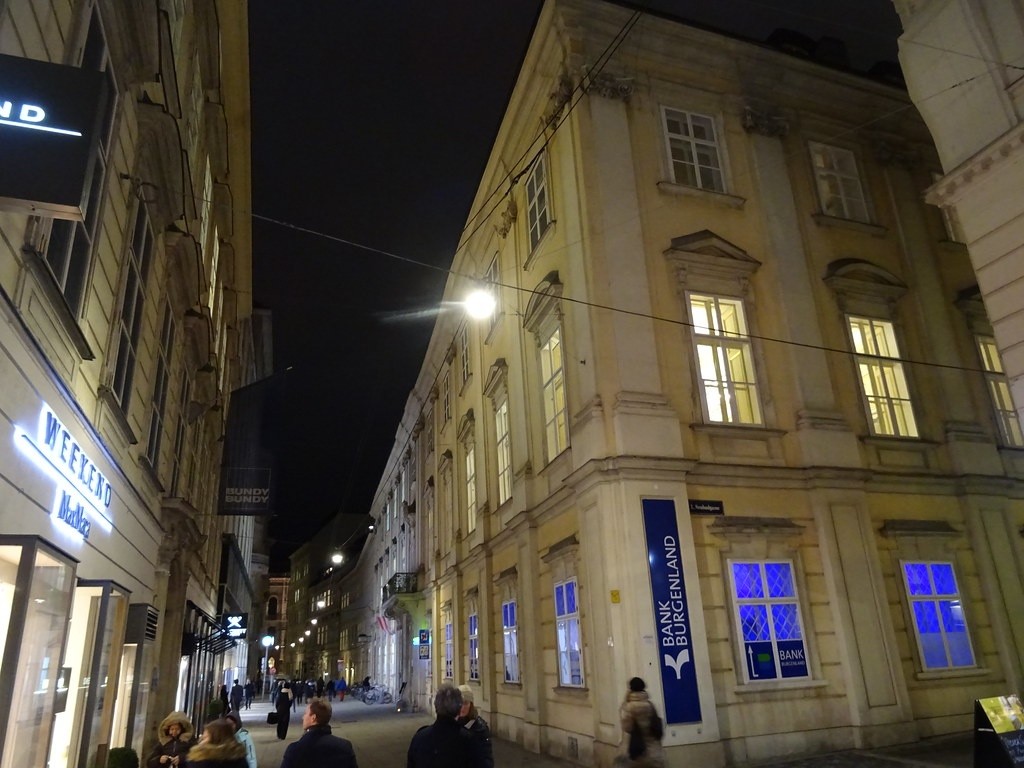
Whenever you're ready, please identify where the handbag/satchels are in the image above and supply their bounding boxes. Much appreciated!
[267,712,278,724]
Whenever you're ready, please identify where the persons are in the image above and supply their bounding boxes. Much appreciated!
[220,676,263,718]
[362,675,371,704]
[184,719,248,768]
[279,698,359,768]
[407,681,495,768]
[620,677,664,768]
[223,710,257,767]
[145,710,197,768]
[275,682,293,740]
[270,675,347,713]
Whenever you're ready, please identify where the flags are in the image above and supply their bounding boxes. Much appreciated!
[376,616,394,635]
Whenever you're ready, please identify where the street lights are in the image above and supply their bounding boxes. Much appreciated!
[262,636,272,703]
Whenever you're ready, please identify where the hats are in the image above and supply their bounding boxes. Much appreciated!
[458,685,474,704]
[630,677,645,691]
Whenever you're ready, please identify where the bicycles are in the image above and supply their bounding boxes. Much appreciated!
[345,682,393,705]
